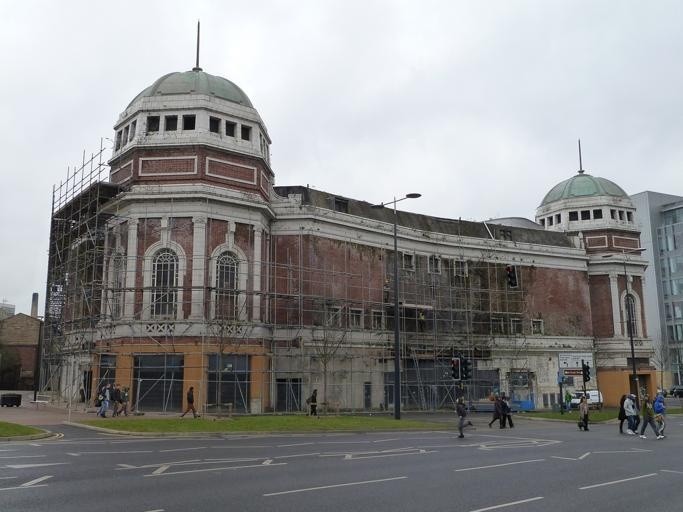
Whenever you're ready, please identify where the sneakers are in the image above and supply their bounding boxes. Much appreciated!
[657,435,665,440]
[640,435,646,439]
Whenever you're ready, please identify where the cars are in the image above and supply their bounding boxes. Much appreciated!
[656,385,682,398]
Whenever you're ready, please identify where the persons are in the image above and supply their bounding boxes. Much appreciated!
[311,389,318,416]
[489,397,503,428]
[578,397,589,431]
[456,398,472,437]
[565,391,572,413]
[96,383,130,417]
[618,393,666,440]
[181,387,198,419]
[501,396,514,428]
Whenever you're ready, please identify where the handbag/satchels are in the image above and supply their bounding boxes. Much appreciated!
[578,421,585,427]
[655,414,663,421]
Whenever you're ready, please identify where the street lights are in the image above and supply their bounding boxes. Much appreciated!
[370,191,421,421]
[32,315,43,402]
[600,247,647,410]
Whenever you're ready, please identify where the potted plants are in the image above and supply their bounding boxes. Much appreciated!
[564,390,573,410]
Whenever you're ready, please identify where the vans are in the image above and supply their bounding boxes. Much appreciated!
[568,389,603,409]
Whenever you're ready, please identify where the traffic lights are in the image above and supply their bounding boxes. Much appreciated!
[461,356,472,380]
[582,365,590,381]
[505,264,518,289]
[450,358,461,381]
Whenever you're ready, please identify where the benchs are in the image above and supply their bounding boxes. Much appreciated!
[29,395,54,410]
[0,394,21,407]
[577,404,601,410]
